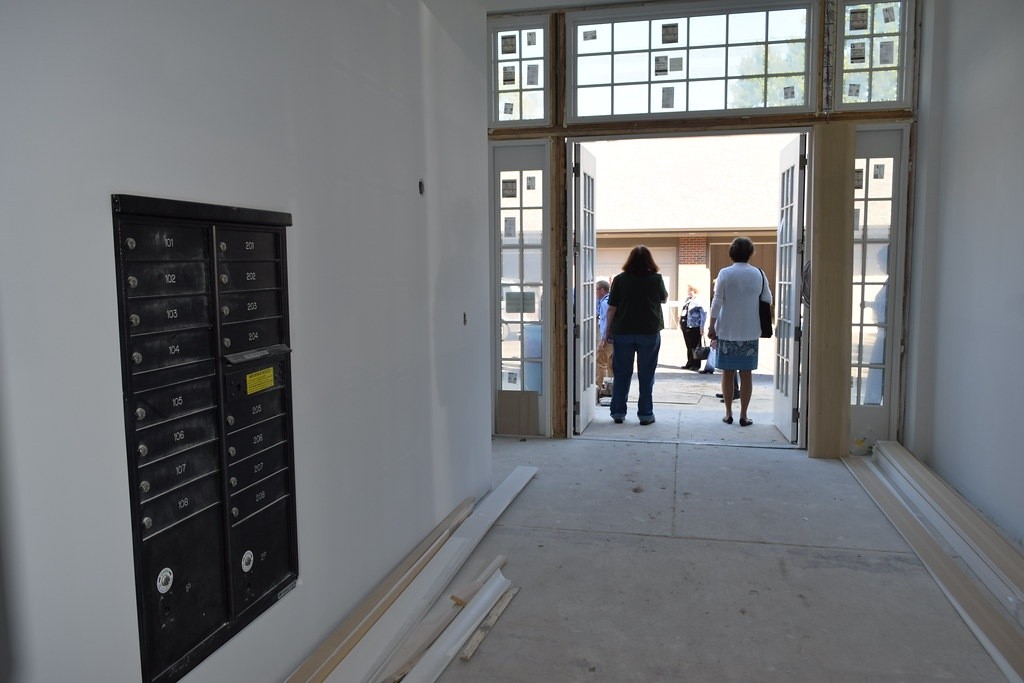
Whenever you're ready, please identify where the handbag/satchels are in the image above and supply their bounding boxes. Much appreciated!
[692,334,710,360]
[758,268,773,338]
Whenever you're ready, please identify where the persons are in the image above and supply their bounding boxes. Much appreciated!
[604,247,668,426]
[681,283,707,370]
[866,243,890,402]
[700,331,717,374]
[595,281,613,405]
[708,238,774,425]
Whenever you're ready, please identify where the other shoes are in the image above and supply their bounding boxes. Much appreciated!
[699,370,713,374]
[615,417,625,423]
[681,365,689,369]
[720,393,740,403]
[640,417,655,425]
[722,417,733,424]
[739,418,753,426]
[716,390,740,398]
[600,389,612,397]
[690,366,699,370]
